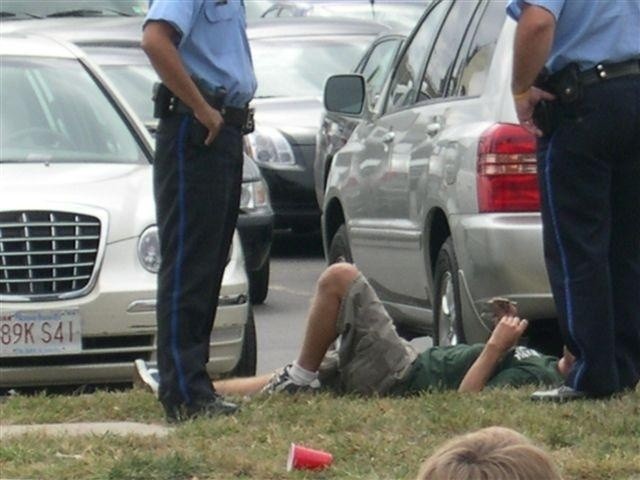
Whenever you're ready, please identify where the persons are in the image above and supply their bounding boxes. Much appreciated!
[417,425,560,480]
[133,261,577,402]
[504,0,640,404]
[139,0,259,423]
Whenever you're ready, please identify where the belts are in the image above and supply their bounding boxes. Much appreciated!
[581,59,640,89]
[174,100,254,135]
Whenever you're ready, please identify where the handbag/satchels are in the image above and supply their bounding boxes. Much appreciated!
[151,82,173,119]
[532,100,559,136]
[547,66,581,105]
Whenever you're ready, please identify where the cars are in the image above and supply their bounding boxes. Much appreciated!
[315,32,448,215]
[321,0,560,356]
[234,18,408,240]
[0,33,258,389]
[1,16,275,305]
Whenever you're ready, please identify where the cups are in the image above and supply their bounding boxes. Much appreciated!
[285,444,332,471]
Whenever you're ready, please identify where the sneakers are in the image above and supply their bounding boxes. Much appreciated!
[134,358,160,399]
[531,384,585,401]
[166,398,239,424]
[261,363,321,397]
